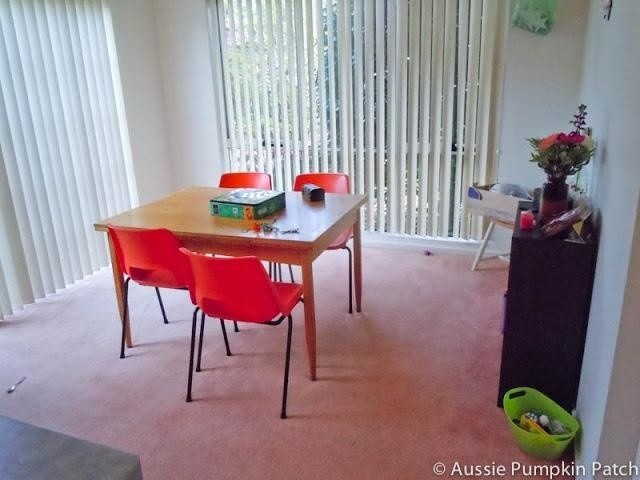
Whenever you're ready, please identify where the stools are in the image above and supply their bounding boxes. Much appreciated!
[471,219,514,274]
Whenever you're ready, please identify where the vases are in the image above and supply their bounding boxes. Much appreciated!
[539,186,568,223]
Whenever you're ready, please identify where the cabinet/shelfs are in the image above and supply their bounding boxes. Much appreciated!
[497,200,595,418]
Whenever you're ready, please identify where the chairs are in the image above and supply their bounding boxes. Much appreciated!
[212,173,294,283]
[177,248,304,420]
[108,225,239,357]
[271,173,355,313]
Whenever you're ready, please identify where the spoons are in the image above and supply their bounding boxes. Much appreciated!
[7,377,28,392]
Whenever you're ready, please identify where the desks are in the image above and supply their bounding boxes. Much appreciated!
[0,417,144,480]
[93,186,369,381]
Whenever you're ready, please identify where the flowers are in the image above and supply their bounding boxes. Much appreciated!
[525,104,598,185]
